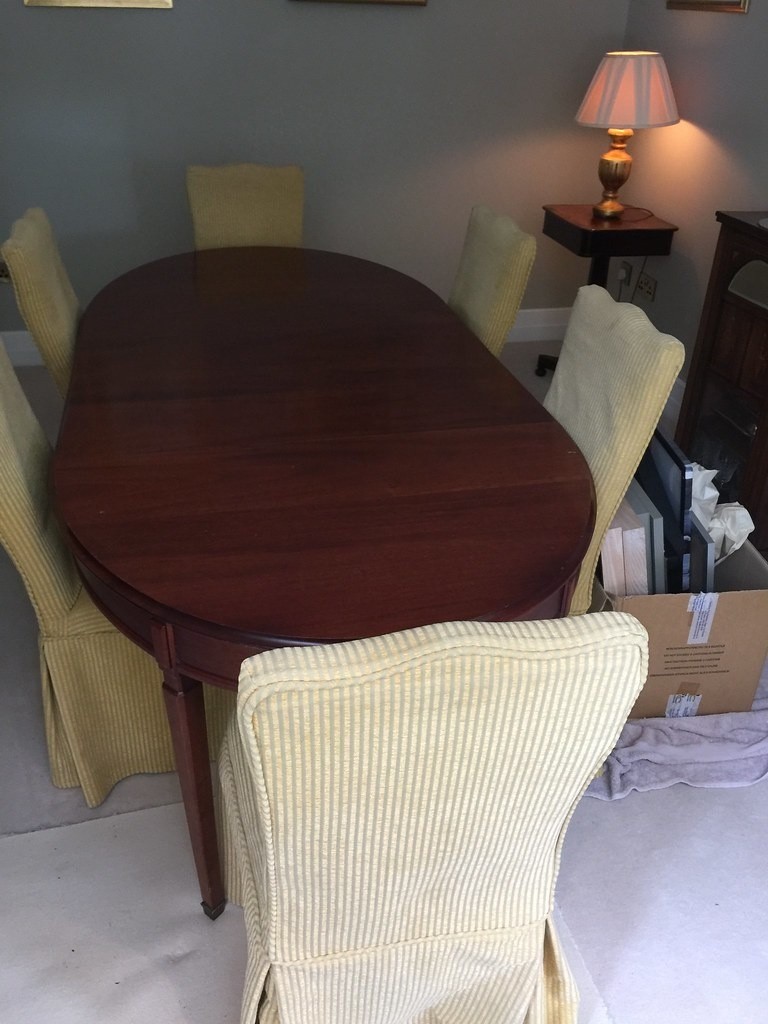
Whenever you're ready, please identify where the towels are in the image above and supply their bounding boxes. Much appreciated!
[582,696,768,802]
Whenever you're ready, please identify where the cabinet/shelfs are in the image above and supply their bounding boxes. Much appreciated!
[673,210,768,563]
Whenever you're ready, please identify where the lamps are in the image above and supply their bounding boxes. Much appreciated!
[573,51,680,219]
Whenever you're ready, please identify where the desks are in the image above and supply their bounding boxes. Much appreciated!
[52,246,597,918]
[536,204,678,376]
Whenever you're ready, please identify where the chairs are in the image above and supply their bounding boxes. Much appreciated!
[541,284,685,612]
[218,613,648,1024]
[0,340,238,809]
[186,165,305,249]
[0,206,83,400]
[447,204,538,355]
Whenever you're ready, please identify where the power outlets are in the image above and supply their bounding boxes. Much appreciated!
[636,272,656,302]
[616,261,633,286]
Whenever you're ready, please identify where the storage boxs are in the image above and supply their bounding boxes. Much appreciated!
[589,538,768,719]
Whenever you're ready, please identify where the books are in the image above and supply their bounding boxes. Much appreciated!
[594,425,715,596]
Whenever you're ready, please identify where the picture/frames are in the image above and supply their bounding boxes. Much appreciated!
[666,0,750,14]
